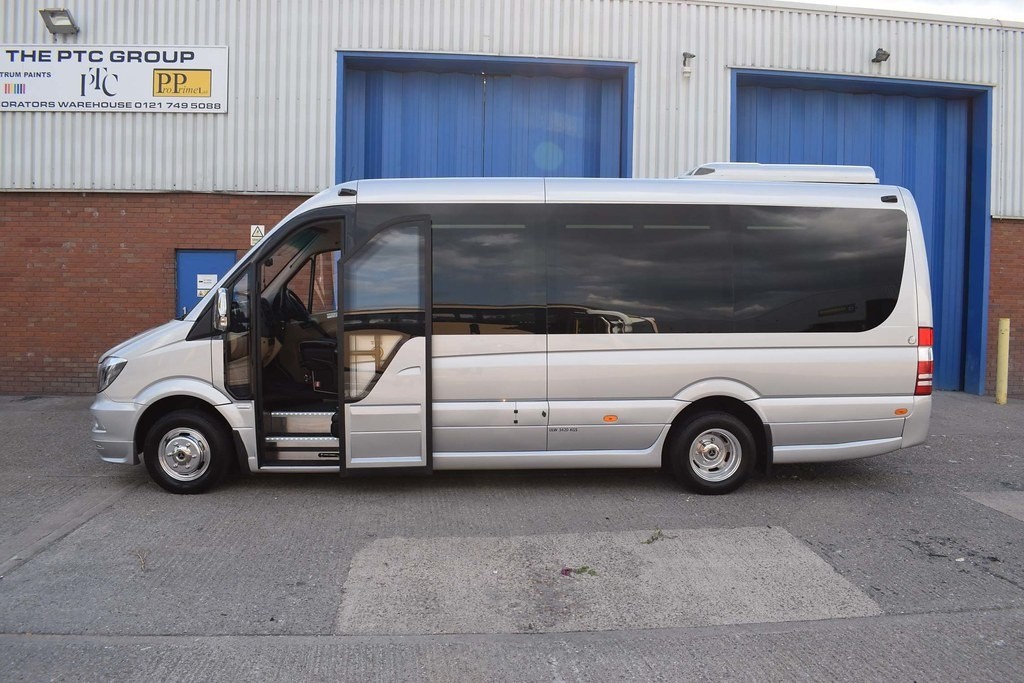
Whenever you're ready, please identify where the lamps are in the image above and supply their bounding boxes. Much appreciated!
[871,49,890,62]
[38,8,79,35]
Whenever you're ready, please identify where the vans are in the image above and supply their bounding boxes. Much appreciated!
[92,161,934,498]
